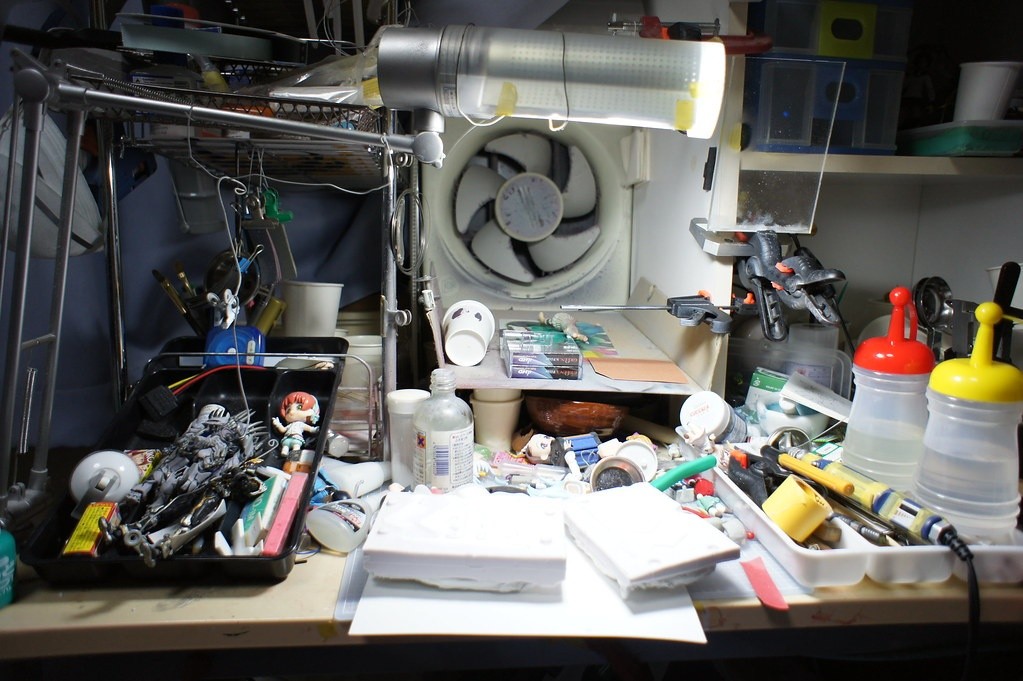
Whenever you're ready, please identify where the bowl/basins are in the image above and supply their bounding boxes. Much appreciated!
[757,391,829,437]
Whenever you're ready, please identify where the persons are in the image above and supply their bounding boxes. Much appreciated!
[524,434,574,466]
[272,392,320,457]
[539,311,588,343]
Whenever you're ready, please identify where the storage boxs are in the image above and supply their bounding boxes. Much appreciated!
[497,317,583,380]
[741,0,1023,157]
[19,337,350,589]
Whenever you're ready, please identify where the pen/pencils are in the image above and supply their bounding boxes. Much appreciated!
[177,271,197,298]
[151,268,207,341]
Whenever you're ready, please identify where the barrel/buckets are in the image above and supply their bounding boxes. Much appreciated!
[281,280,344,337]
[335,329,382,387]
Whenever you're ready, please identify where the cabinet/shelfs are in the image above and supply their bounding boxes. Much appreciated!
[88,0,403,459]
[630,0,1023,426]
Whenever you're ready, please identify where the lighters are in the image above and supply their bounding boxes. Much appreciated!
[282,449,316,476]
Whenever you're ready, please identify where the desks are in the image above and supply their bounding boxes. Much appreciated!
[0,485,1023,663]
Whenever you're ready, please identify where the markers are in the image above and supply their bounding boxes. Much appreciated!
[256,298,286,337]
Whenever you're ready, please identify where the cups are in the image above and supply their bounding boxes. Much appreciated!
[953,61,1023,121]
[680,391,747,443]
[441,299,495,366]
[386,389,431,490]
[469,389,524,452]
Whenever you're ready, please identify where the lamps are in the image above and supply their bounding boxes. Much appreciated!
[0,23,729,532]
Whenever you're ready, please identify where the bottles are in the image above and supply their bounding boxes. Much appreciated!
[194,55,230,93]
[413,369,474,494]
[306,498,371,552]
[841,286,1023,528]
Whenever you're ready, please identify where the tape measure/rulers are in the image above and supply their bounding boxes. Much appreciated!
[203,323,265,370]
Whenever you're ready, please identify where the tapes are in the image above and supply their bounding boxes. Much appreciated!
[762,473,833,543]
